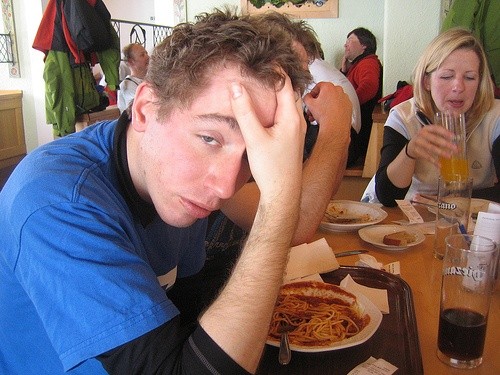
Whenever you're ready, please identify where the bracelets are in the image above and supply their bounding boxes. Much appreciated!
[405,142,416,159]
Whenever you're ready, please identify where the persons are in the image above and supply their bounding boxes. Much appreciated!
[360,28,500,207]
[93,19,383,305]
[0,9,308,375]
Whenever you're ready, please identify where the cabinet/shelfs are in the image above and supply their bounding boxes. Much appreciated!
[1,89,28,159]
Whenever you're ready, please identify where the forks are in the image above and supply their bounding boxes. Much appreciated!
[410,200,442,212]
[276,320,296,365]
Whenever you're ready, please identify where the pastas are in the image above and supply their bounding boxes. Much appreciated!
[268,293,359,346]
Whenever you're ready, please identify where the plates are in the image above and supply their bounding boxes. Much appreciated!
[266,282,384,353]
[358,224,426,250]
[318,200,387,233]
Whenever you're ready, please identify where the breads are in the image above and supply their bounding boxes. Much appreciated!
[383,230,417,246]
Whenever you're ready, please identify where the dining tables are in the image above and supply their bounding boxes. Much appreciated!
[308,202,500,375]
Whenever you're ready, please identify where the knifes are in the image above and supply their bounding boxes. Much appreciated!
[419,194,463,217]
[334,250,365,257]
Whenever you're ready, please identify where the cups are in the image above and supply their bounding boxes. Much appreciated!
[433,178,473,260]
[437,233,497,371]
[433,110,470,191]
